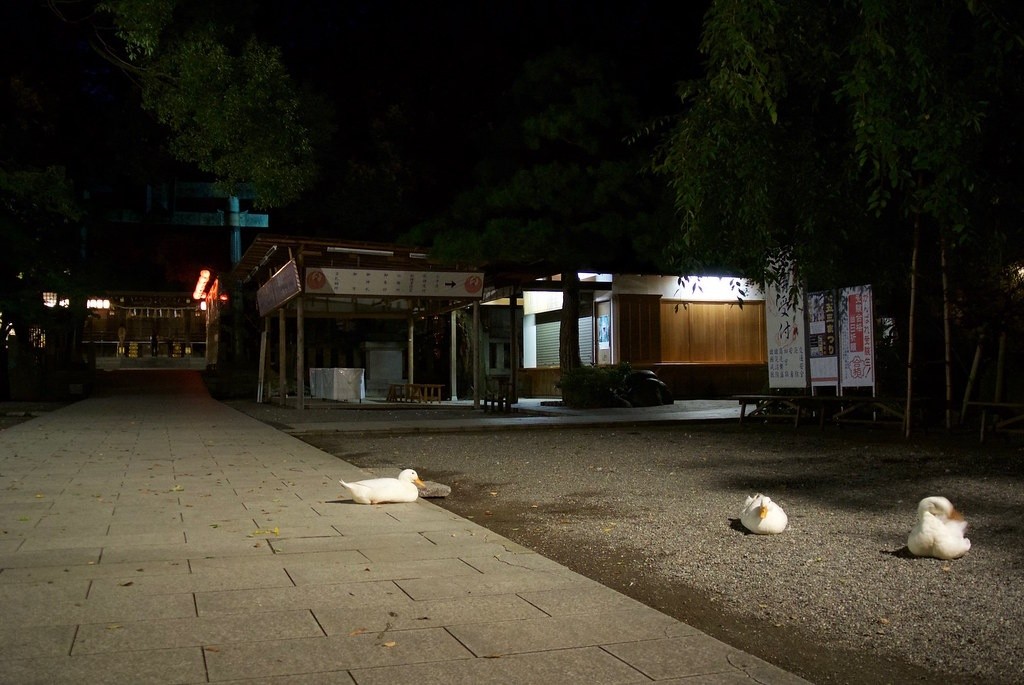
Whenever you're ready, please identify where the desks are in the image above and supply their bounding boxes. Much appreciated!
[309,368,366,404]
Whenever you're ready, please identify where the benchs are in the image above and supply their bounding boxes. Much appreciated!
[385,384,447,403]
[733,395,929,438]
[966,401,1024,442]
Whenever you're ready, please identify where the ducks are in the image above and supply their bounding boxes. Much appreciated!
[740,493,788,534]
[339,469,425,505]
[907,496,971,559]
